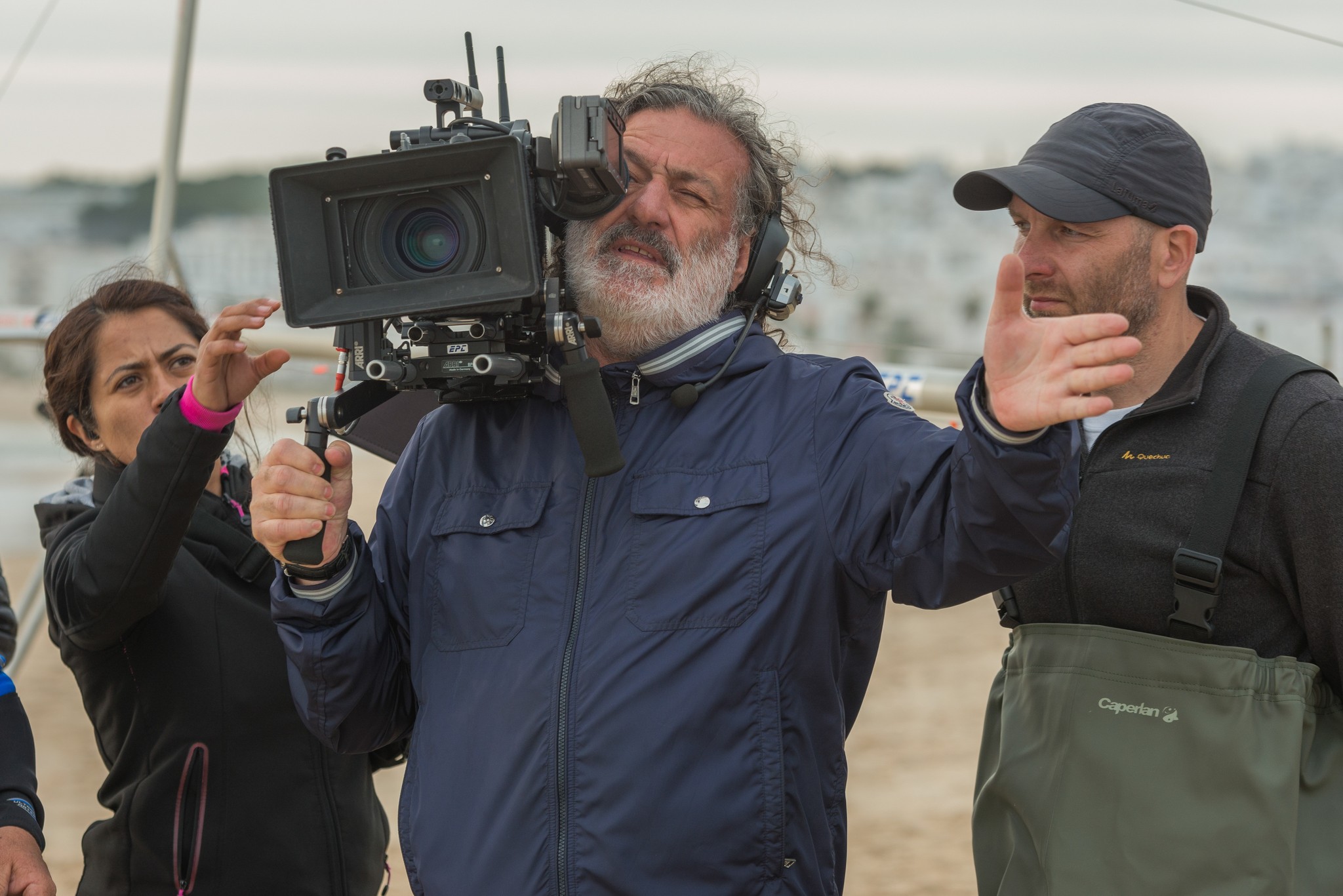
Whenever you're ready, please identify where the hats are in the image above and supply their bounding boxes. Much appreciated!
[953,103,1212,254]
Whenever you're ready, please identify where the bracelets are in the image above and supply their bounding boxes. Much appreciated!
[282,527,355,581]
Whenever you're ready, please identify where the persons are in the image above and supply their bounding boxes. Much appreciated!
[248,53,1142,896]
[0,279,391,896]
[954,104,1342,895]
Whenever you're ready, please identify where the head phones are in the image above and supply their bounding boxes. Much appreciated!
[730,170,804,323]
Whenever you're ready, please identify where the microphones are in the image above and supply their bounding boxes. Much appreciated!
[670,291,765,407]
